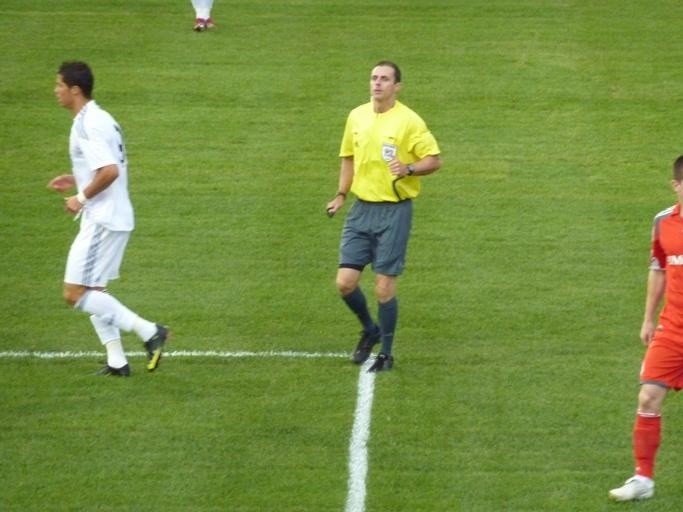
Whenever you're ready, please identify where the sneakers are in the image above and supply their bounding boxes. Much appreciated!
[95,364,130,378]
[351,325,380,364]
[194,17,217,31]
[144,324,166,370]
[610,475,655,501]
[367,352,393,372]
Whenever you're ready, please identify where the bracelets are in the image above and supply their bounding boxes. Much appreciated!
[76,192,87,205]
[335,191,346,200]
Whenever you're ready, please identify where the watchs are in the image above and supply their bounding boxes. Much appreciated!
[406,164,415,176]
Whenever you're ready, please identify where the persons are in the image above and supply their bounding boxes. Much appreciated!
[43,61,172,378]
[324,60,443,374]
[190,0,216,32]
[605,154,682,502]
